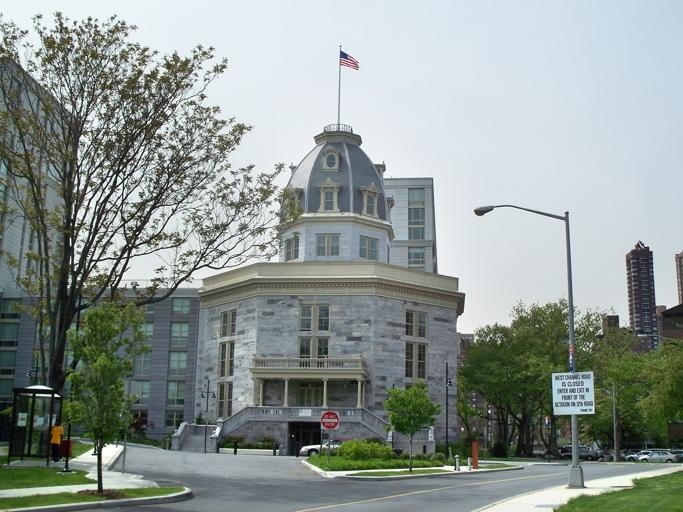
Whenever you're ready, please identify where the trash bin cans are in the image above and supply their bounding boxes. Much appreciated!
[279,445,287,456]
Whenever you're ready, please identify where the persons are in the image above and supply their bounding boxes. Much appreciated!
[51,419,64,463]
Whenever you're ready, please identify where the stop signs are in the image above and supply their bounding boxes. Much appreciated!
[320,412,339,428]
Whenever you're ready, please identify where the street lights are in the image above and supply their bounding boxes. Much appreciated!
[637,333,669,340]
[475,204,585,489]
[198,380,216,453]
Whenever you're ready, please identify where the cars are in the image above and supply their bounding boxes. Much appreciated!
[558,443,682,463]
[299,439,343,458]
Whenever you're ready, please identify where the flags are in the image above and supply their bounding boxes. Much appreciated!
[340,50,360,71]
